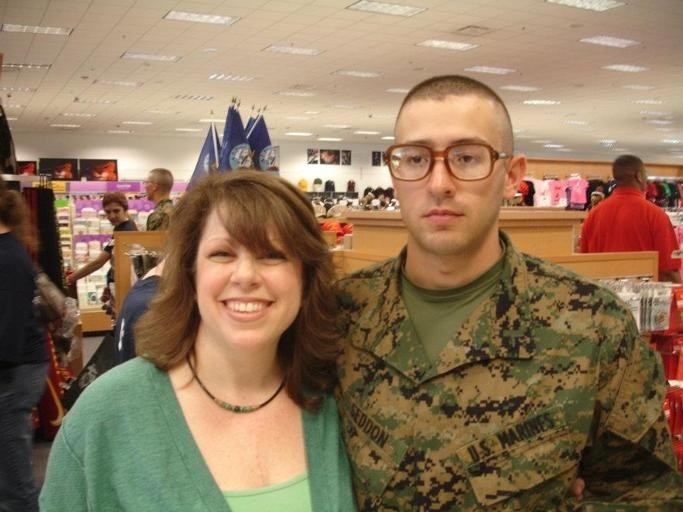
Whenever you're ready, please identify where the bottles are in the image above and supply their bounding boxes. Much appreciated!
[347,179,355,191]
[312,178,334,191]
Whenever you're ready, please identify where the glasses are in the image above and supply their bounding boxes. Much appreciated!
[380,141,516,182]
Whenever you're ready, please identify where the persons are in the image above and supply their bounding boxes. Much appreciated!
[114,250,170,365]
[37,169,585,512]
[0,190,66,512]
[323,76,683,512]
[66,168,174,313]
[581,155,683,283]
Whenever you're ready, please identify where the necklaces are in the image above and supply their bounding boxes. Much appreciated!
[185,351,285,412]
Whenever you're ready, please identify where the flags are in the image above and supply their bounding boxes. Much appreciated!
[186,108,278,186]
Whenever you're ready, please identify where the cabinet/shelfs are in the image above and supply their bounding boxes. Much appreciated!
[58,206,152,334]
